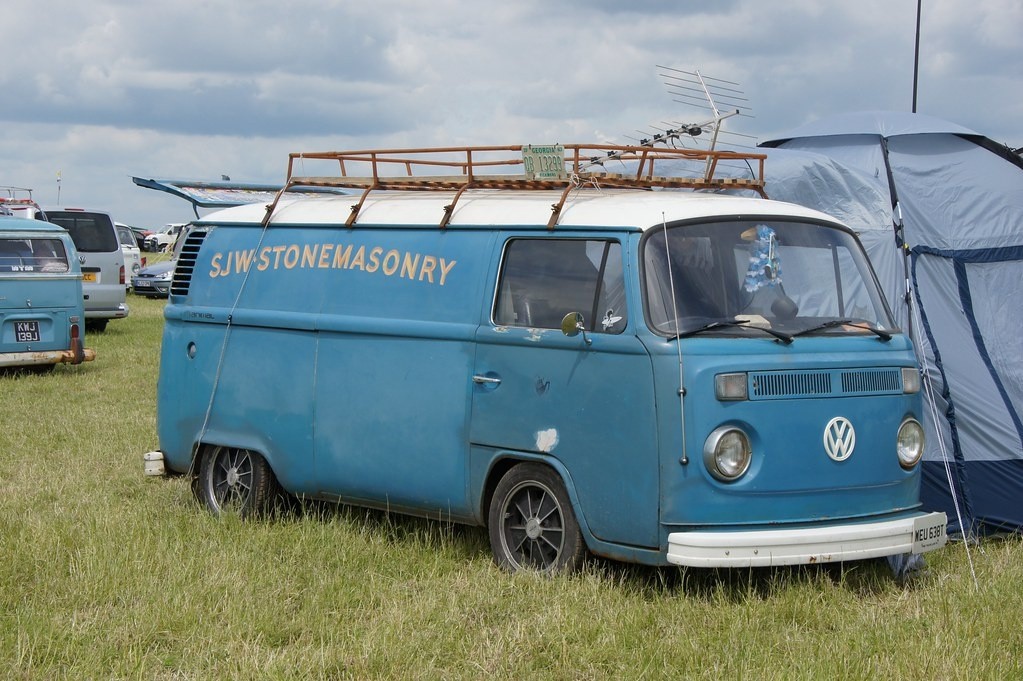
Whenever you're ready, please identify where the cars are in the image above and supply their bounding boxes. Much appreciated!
[133,259,178,299]
[132,230,147,250]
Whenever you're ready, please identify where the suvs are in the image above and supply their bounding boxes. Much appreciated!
[144,222,190,254]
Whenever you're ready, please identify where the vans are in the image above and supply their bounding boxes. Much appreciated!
[131,174,953,584]
[34,207,130,334]
[114,221,142,290]
[0,216,97,375]
